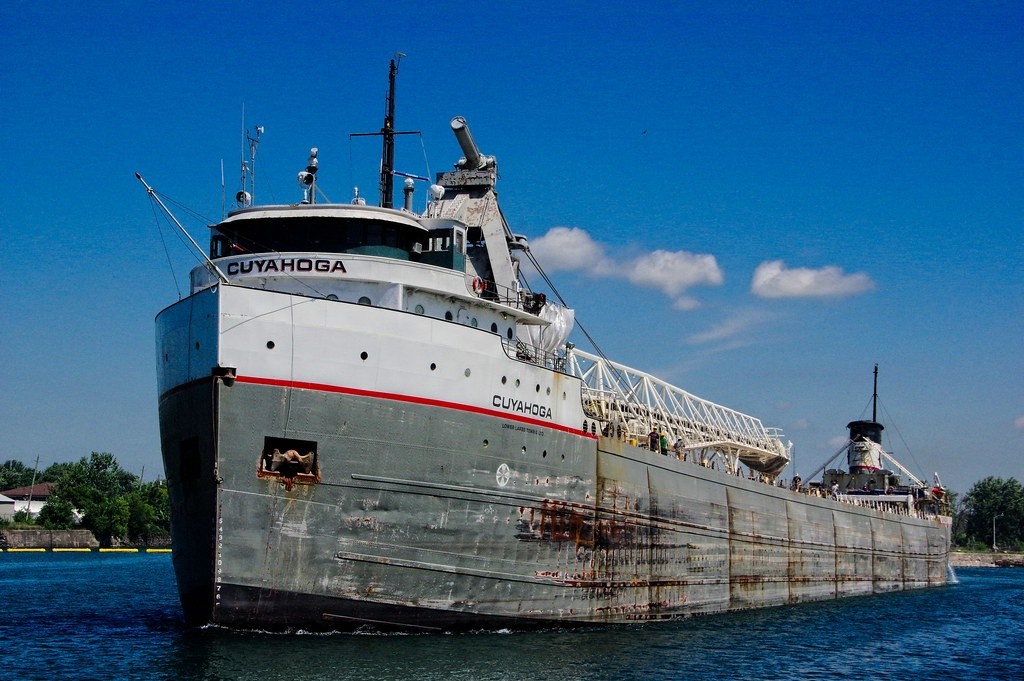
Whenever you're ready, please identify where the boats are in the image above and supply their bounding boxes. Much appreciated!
[133,51,955,632]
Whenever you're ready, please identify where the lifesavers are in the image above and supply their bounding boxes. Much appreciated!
[540,295,544,301]
[864,484,867,491]
[473,276,484,294]
[888,486,894,493]
[947,496,949,503]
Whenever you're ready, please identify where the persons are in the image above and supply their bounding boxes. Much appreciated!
[648,426,685,461]
[791,472,802,492]
[759,470,783,488]
[830,479,839,502]
